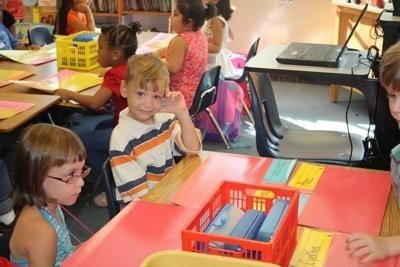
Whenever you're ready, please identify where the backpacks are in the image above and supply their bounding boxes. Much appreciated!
[227,54,251,114]
[191,81,244,142]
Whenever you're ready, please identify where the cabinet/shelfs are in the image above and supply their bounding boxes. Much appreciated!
[88,0,171,26]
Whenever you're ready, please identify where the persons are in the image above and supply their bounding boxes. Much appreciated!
[344,40,400,267]
[0,1,241,234]
[8,122,89,266]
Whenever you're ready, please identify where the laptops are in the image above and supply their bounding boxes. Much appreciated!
[276,3,368,67]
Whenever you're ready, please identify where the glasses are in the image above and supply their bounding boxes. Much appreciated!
[46,166,91,184]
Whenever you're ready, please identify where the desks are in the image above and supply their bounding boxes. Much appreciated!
[330,0,383,101]
[377,8,399,56]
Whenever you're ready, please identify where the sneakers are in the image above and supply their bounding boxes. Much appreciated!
[93,191,108,207]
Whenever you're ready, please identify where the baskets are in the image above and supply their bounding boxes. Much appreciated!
[181,180,299,267]
[56,31,100,71]
